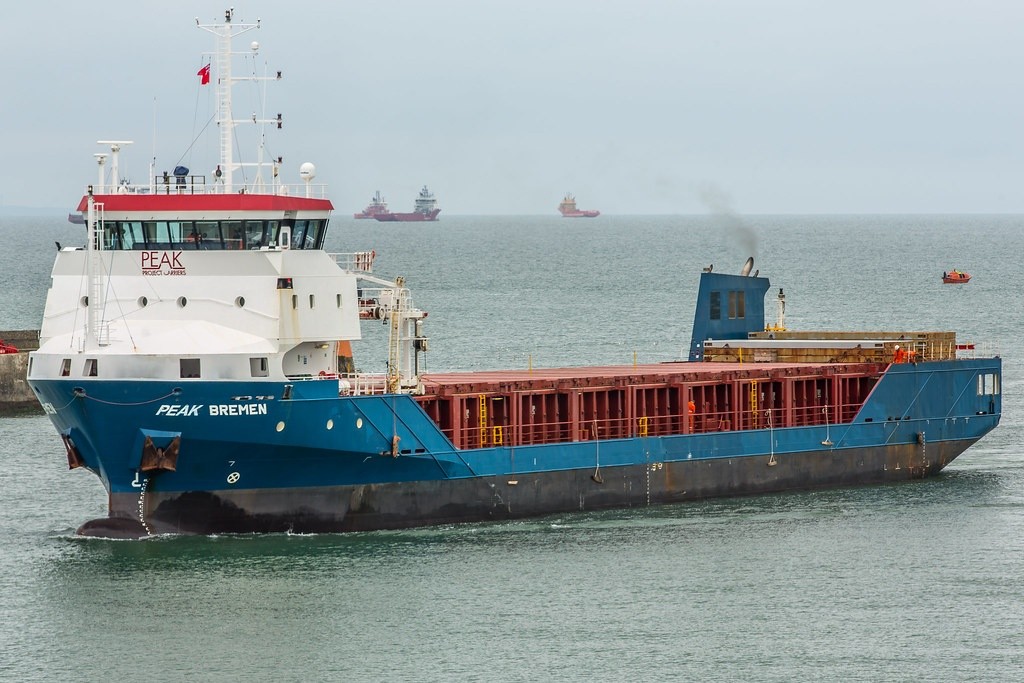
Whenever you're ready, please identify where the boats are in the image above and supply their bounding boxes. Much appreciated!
[941,269,972,284]
[353,192,390,220]
[557,197,601,217]
[373,185,441,221]
[27,6,1003,539]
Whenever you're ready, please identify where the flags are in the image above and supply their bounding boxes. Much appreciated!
[197,62,210,85]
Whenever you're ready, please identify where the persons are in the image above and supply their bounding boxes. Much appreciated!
[232,230,240,249]
[688,400,696,434]
[187,228,199,241]
[893,345,917,364]
